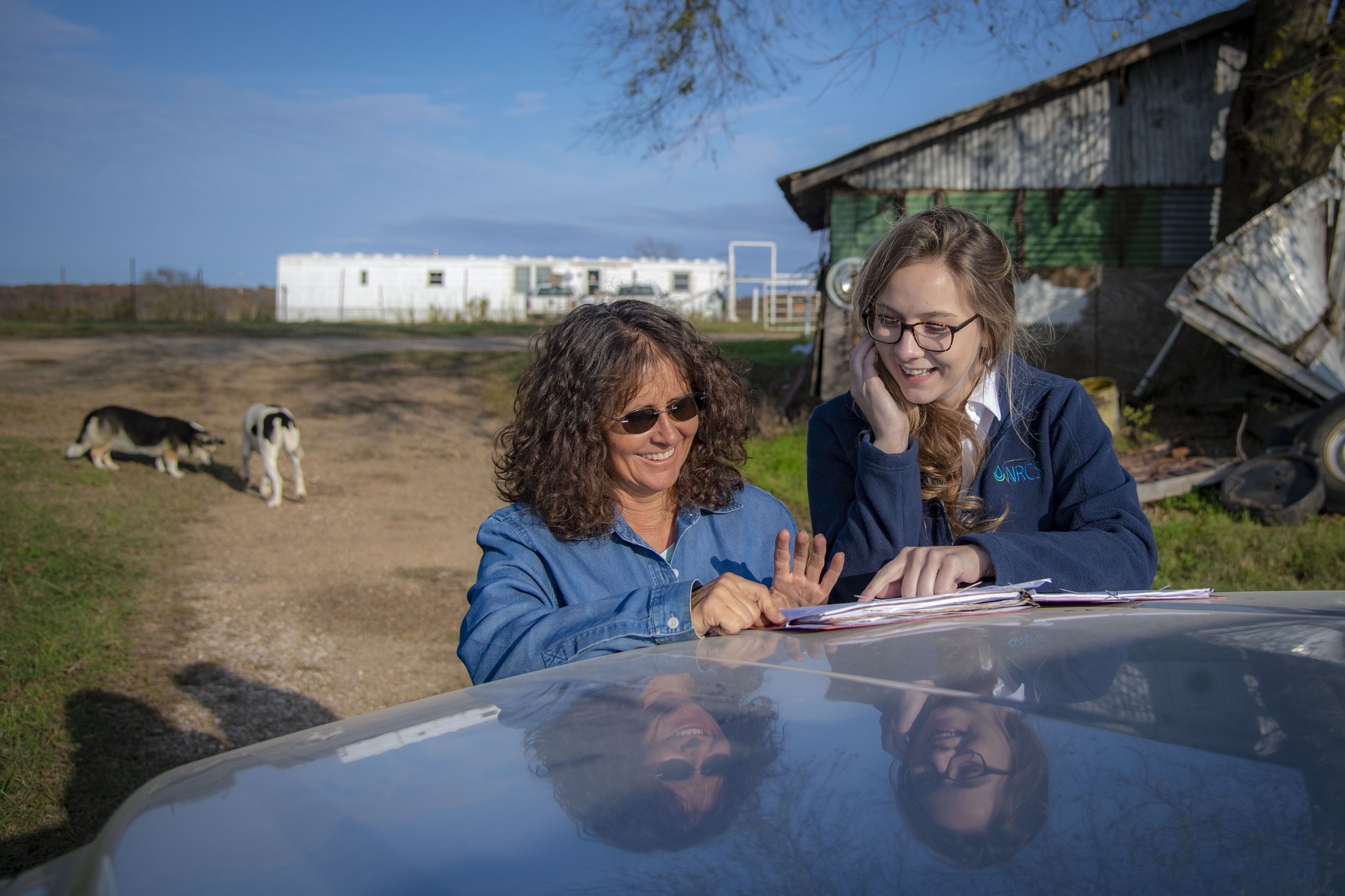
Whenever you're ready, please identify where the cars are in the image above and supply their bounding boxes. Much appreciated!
[527,283,580,322]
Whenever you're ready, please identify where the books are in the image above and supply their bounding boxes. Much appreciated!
[778,578,1211,630]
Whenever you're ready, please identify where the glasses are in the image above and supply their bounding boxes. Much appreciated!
[651,755,732,782]
[903,751,1016,794]
[601,393,708,435]
[862,304,980,352]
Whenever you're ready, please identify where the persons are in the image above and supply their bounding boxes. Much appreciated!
[825,617,1128,870]
[467,630,838,854]
[456,299,845,686]
[806,207,1159,605]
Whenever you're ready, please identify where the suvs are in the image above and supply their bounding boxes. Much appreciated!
[609,283,670,310]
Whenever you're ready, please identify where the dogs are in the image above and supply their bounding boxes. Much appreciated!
[242,402,308,507]
[65,406,225,480]
